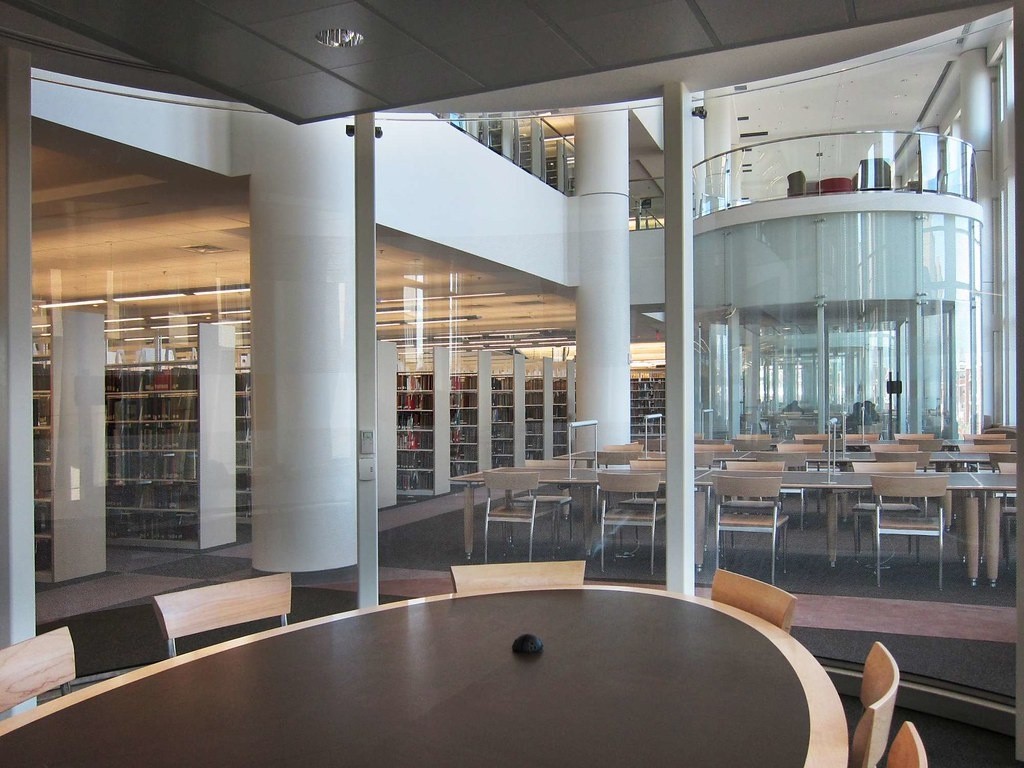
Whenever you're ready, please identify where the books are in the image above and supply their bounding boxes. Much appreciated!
[628,372,668,436]
[106,368,201,541]
[397,373,569,491]
[236,372,252,517]
[33,360,52,571]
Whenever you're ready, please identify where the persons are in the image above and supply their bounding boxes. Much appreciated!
[846,401,871,433]
[862,401,880,425]
[781,400,805,413]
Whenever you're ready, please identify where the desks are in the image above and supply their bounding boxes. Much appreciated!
[779,440,952,472]
[0,583,848,768]
[949,451,1017,531]
[553,450,750,514]
[693,470,982,587]
[946,440,975,472]
[449,466,710,575]
[740,450,956,533]
[971,472,1016,588]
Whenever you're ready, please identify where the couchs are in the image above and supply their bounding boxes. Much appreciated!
[787,158,893,197]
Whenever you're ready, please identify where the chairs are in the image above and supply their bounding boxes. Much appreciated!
[0,626,76,714]
[445,411,1024,768]
[151,572,293,658]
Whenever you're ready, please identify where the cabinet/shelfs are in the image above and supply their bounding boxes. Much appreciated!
[435,113,574,197]
[32,308,666,585]
